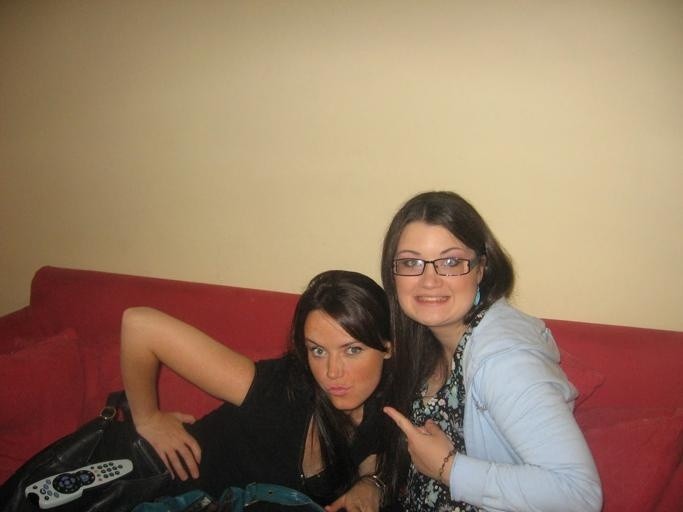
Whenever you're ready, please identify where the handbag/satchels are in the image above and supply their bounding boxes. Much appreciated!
[0,385,183,512]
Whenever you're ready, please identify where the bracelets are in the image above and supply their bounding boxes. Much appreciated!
[360,474,389,498]
[435,448,457,486]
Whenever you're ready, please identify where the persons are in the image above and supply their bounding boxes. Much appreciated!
[117,269,395,512]
[378,189,603,511]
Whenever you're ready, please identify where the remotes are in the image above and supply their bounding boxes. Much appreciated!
[24,456,136,510]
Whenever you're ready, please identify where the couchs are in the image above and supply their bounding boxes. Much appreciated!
[0,265,683,512]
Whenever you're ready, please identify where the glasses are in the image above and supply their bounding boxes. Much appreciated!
[391,255,482,277]
